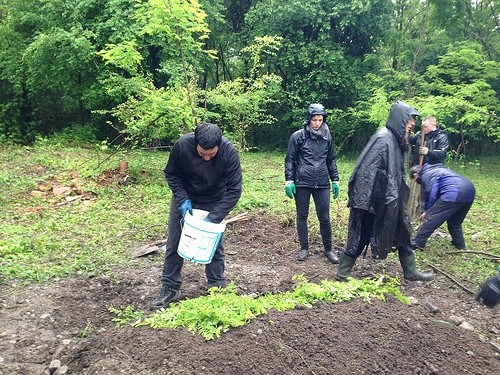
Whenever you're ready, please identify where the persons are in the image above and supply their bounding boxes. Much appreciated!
[149,124,242,310]
[409,163,475,251]
[285,104,339,265]
[409,116,449,166]
[475,273,500,309]
[334,103,435,282]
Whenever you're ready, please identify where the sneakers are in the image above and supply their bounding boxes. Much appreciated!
[150,284,181,311]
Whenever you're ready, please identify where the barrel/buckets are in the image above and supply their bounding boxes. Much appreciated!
[176,208,227,265]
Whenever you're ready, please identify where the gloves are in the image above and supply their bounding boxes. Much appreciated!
[476,276,500,308]
[178,199,193,218]
[285,181,296,199]
[203,217,212,223]
[332,181,339,199]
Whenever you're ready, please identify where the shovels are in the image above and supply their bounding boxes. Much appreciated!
[132,212,252,257]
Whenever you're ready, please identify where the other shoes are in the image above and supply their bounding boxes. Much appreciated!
[410,239,423,251]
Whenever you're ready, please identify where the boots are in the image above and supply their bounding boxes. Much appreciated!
[334,252,356,282]
[399,253,434,281]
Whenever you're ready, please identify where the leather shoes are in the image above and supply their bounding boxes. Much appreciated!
[299,250,308,260]
[325,251,340,264]
